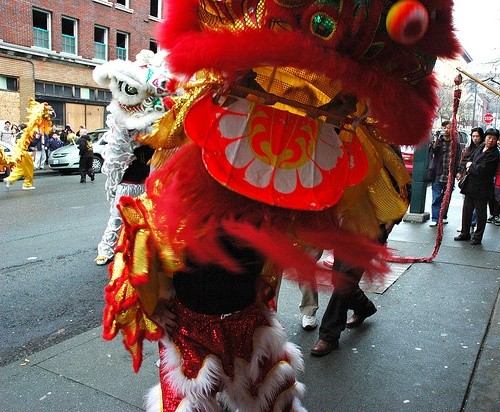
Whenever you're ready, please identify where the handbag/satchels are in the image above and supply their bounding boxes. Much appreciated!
[458,175,467,190]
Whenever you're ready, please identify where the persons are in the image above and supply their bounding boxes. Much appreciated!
[425,121,461,227]
[456,127,485,234]
[2,123,40,190]
[32,133,49,170]
[4,121,10,131]
[48,126,75,152]
[273,246,323,329]
[454,128,500,245]
[487,171,500,226]
[311,145,412,355]
[74,129,95,183]
[93,142,156,265]
[76,126,83,137]
[11,125,21,133]
[143,243,309,412]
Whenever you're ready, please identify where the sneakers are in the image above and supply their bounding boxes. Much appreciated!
[470,237,482,245]
[487,215,498,223]
[454,234,470,240]
[495,217,500,225]
[301,315,316,329]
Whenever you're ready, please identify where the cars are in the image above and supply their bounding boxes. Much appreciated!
[0,140,40,181]
[401,129,469,176]
[48,129,109,172]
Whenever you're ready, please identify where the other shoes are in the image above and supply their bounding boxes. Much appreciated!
[457,228,461,232]
[40,165,44,169]
[443,218,448,224]
[3,178,9,190]
[96,255,107,265]
[91,173,94,181]
[470,226,474,234]
[429,221,437,227]
[80,179,86,183]
[22,185,35,190]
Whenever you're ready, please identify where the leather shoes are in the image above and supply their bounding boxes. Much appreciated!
[311,339,339,355]
[346,302,378,327]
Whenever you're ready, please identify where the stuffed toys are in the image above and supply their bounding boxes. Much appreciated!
[0,96,56,172]
[103,0,462,374]
[93,48,181,204]
